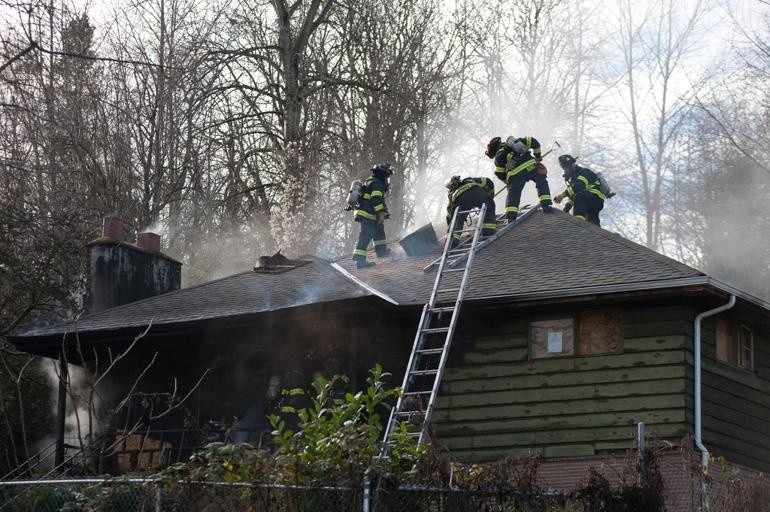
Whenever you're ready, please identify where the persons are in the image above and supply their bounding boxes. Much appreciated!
[444,175,497,250]
[349,162,394,270]
[553,154,606,229]
[485,135,554,228]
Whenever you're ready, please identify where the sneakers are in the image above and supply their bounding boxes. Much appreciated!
[503,218,515,224]
[376,248,391,258]
[356,261,375,268]
[542,206,554,213]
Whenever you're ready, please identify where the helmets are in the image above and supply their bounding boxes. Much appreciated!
[558,155,576,167]
[369,163,394,178]
[485,137,501,158]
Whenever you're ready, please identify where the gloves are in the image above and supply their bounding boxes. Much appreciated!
[553,191,568,204]
[534,153,543,162]
[563,203,572,213]
[377,210,391,224]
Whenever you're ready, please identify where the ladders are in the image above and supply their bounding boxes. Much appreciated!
[379,203,489,468]
